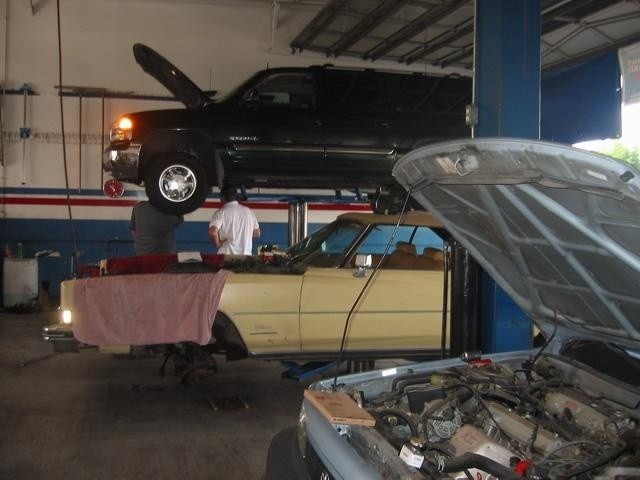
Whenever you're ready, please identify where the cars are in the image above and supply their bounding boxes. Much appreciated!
[41,212,452,361]
[265,136,640,480]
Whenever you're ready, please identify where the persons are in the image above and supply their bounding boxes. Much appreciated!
[130,199,184,254]
[209,184,261,255]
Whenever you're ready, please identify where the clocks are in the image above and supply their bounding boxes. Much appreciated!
[104,180,125,198]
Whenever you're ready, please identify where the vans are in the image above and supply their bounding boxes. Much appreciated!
[103,40,472,215]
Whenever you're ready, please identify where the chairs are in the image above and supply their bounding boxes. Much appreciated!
[412,247,445,270]
[384,242,417,268]
